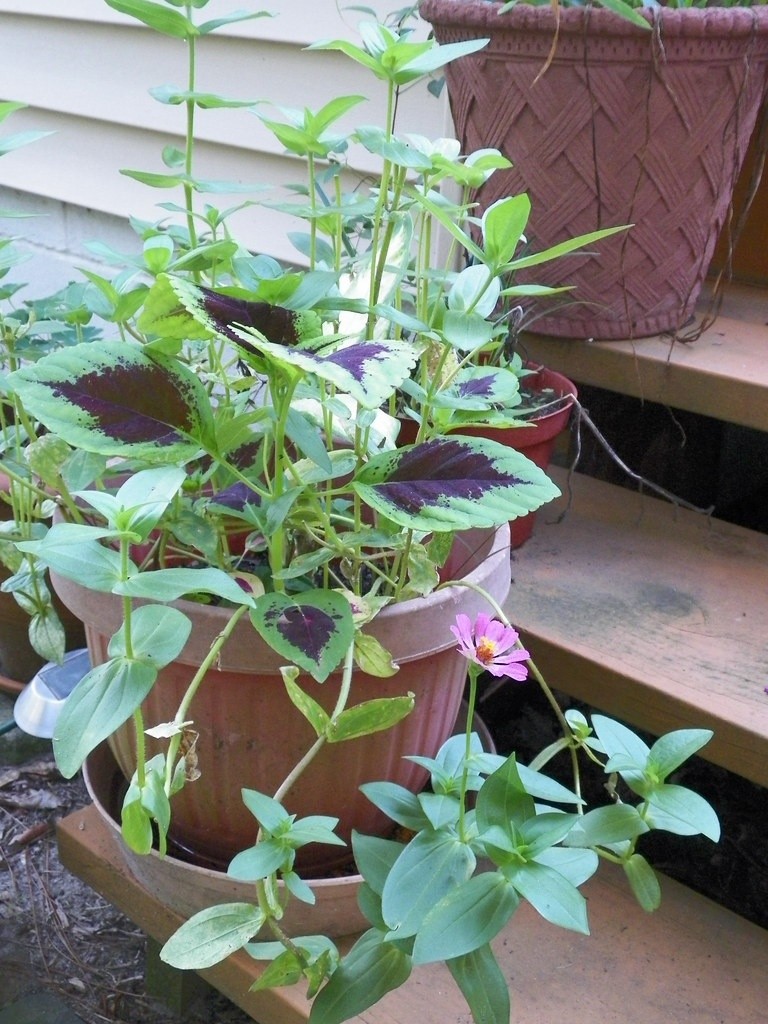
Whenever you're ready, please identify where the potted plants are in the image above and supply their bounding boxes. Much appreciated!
[0,2,768,1024]
